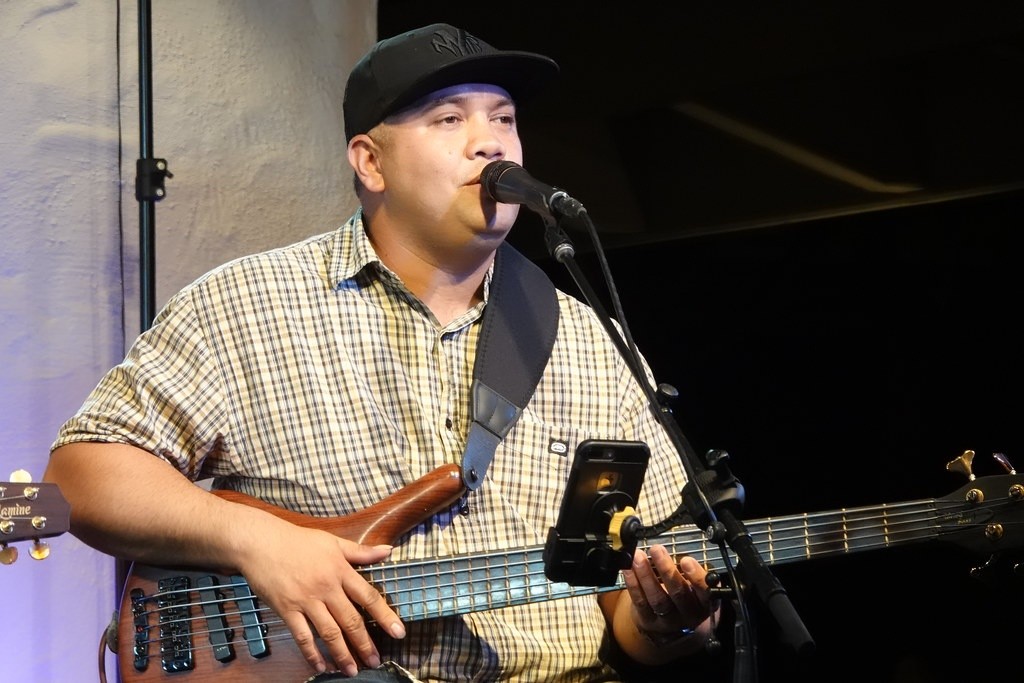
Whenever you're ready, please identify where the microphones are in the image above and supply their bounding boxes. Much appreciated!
[480,160,588,219]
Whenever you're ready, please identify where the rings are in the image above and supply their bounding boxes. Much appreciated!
[653,600,673,617]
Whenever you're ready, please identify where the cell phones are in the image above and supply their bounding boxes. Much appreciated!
[545,439,651,588]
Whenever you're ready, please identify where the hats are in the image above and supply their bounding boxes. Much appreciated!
[343,23,559,146]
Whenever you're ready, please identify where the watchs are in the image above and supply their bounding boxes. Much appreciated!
[637,623,702,650]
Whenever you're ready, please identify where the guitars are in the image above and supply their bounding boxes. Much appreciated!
[106,448,1024,683]
[0,468,74,567]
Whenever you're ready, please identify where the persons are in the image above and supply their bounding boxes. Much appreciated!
[41,24,723,682]
